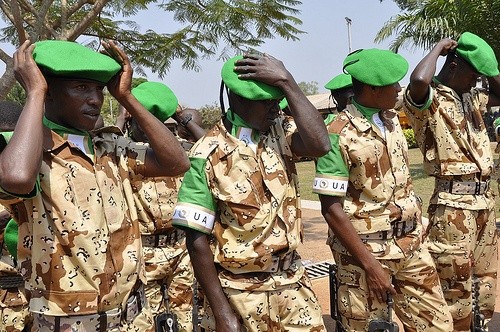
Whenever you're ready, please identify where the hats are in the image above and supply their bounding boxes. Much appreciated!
[31,41,122,85]
[131,82,178,121]
[456,32,499,77]
[343,48,409,86]
[221,55,286,100]
[324,73,353,89]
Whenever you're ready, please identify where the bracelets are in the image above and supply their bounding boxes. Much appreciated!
[180,113,192,126]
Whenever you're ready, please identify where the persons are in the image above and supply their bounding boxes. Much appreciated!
[0,32,500,332]
